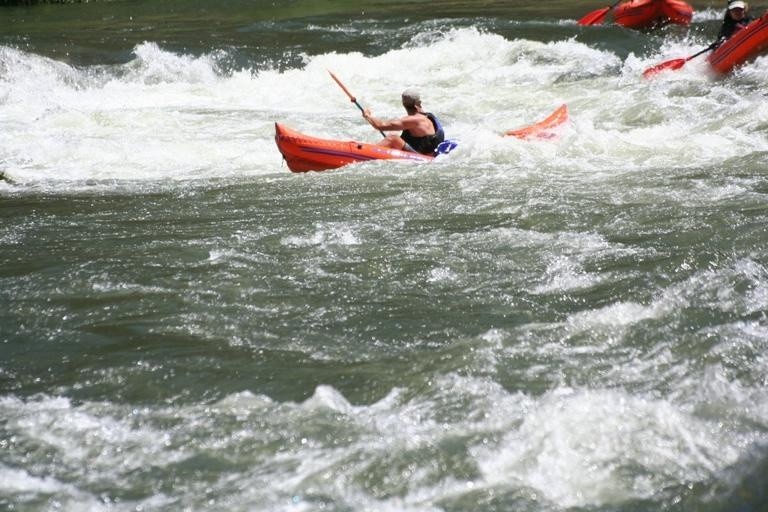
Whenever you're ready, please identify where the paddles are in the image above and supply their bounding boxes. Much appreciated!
[577,0,622,24]
[331,73,385,138]
[643,44,717,81]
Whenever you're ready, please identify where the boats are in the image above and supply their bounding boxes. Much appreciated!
[273,103,569,174]
[704,10,767,73]
[609,0,693,29]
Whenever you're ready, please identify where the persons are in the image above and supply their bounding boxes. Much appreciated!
[364,90,446,155]
[715,1,754,45]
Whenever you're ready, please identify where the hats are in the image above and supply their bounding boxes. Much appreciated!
[728,0,746,11]
